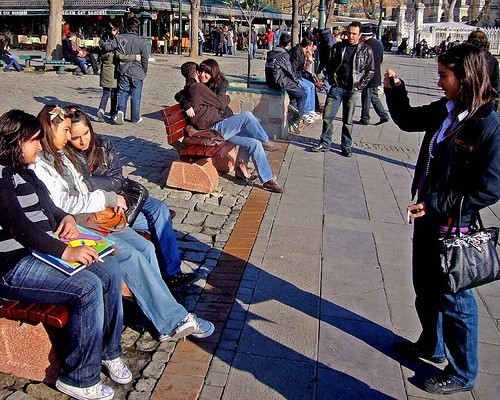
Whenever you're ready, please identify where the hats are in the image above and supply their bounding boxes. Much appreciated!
[360,26,373,35]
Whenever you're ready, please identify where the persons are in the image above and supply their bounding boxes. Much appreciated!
[438,36,452,59]
[180,61,285,194]
[96,21,141,126]
[332,28,351,43]
[264,34,304,134]
[354,28,390,128]
[71,33,102,75]
[99,17,148,124]
[0,109,134,399]
[0,34,24,73]
[175,58,257,182]
[464,31,500,112]
[286,37,321,123]
[62,106,202,291]
[62,33,90,75]
[197,25,258,57]
[311,22,375,157]
[262,25,275,56]
[34,106,216,343]
[384,46,499,394]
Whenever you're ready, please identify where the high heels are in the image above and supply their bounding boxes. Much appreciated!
[235,164,257,181]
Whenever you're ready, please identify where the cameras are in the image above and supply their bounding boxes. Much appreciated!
[388,76,395,89]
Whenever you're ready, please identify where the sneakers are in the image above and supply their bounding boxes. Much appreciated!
[166,273,199,291]
[262,174,284,193]
[311,144,331,152]
[97,109,105,122]
[133,118,143,124]
[406,340,446,363]
[341,146,352,158]
[159,321,195,342]
[288,119,300,134]
[56,378,114,400]
[116,111,124,125]
[102,358,133,384]
[262,140,280,151]
[358,118,369,125]
[375,117,390,125]
[309,111,320,119]
[177,313,214,337]
[300,115,315,124]
[423,373,474,394]
[288,103,299,114]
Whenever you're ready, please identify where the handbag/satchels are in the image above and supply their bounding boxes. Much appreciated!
[183,124,226,146]
[115,179,149,229]
[76,205,126,234]
[437,226,499,294]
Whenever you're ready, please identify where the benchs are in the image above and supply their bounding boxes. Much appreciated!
[17,53,40,73]
[42,58,69,73]
[0,210,176,381]
[163,103,235,193]
